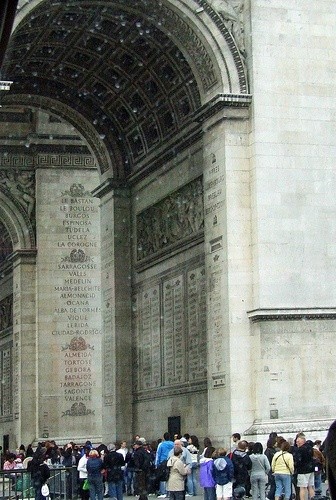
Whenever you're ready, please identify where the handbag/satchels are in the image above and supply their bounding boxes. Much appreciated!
[41,484,50,496]
[157,460,170,482]
[83,480,90,490]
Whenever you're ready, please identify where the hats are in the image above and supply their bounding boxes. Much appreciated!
[133,441,143,445]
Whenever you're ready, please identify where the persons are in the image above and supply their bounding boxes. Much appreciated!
[3,432,328,500]
[324,420,336,500]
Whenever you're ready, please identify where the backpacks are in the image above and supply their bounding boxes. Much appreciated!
[142,451,155,470]
